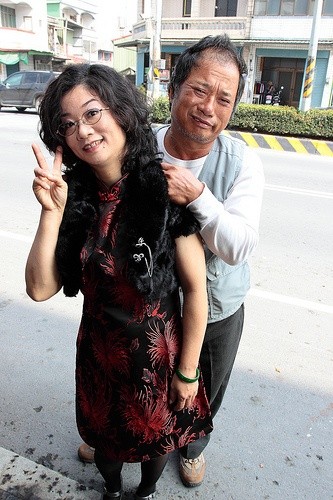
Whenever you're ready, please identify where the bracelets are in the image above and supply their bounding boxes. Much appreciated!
[173,368,201,383]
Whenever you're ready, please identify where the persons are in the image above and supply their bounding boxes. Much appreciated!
[25,64,213,500]
[154,36,263,487]
[264,80,274,97]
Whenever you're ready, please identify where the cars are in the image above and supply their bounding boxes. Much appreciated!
[0,69,63,115]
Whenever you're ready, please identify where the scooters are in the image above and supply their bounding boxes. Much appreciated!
[271,85,284,106]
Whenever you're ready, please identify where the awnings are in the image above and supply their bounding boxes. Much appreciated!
[112,37,150,53]
[0,47,72,65]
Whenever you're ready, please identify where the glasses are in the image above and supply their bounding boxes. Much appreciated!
[55,107,110,137]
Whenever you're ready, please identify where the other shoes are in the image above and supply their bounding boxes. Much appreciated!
[102,479,123,500]
[77,444,95,463]
[179,452,205,487]
[135,484,157,500]
